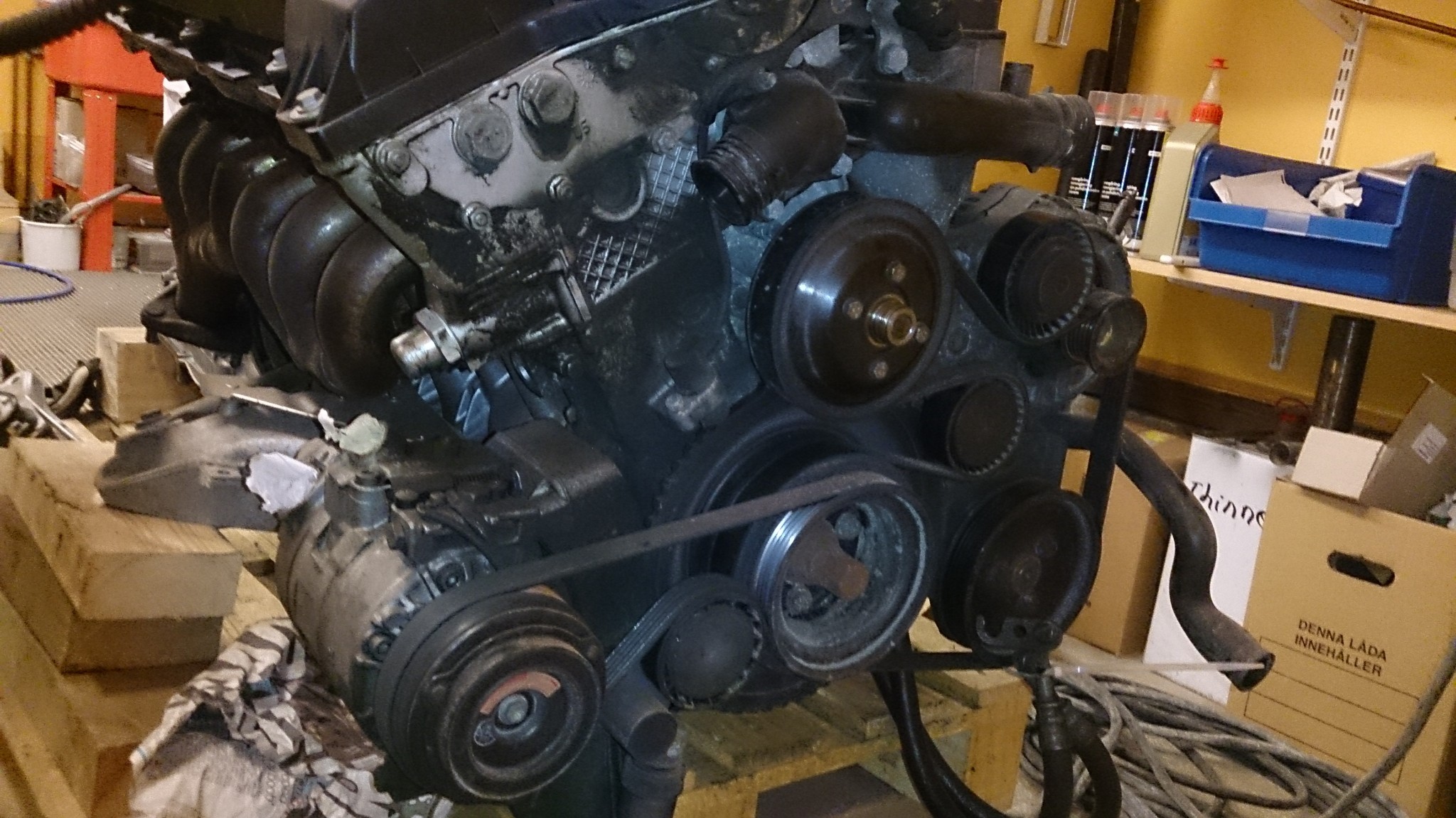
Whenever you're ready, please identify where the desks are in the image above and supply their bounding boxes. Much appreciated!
[1129,258,1455,433]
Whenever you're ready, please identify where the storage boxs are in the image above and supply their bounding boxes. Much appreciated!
[1059,393,1190,659]
[1225,475,1456,818]
[1142,432,1295,709]
[96,327,198,422]
[1292,374,1456,518]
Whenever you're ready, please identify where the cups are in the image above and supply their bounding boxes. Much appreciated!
[19,219,81,269]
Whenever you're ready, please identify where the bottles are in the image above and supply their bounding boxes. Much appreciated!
[1060,57,1229,261]
